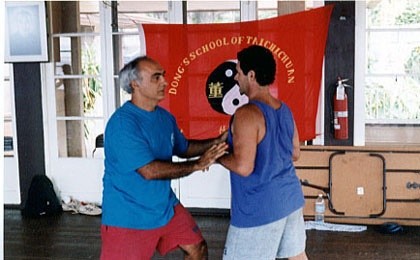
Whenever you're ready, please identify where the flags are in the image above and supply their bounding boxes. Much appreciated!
[134,2,336,142]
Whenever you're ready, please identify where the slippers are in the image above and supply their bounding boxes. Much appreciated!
[374,222,408,235]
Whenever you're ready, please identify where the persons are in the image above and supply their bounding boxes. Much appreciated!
[99,56,230,260]
[200,46,309,260]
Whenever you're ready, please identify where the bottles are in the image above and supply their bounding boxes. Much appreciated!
[314,193,325,223]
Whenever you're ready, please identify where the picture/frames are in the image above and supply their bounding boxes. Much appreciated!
[4,1,51,64]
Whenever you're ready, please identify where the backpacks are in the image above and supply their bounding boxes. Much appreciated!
[20,175,60,218]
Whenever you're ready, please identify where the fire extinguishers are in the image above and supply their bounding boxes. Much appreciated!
[334,79,352,140]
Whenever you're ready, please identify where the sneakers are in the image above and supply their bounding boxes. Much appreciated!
[62,199,80,211]
[76,201,102,215]
